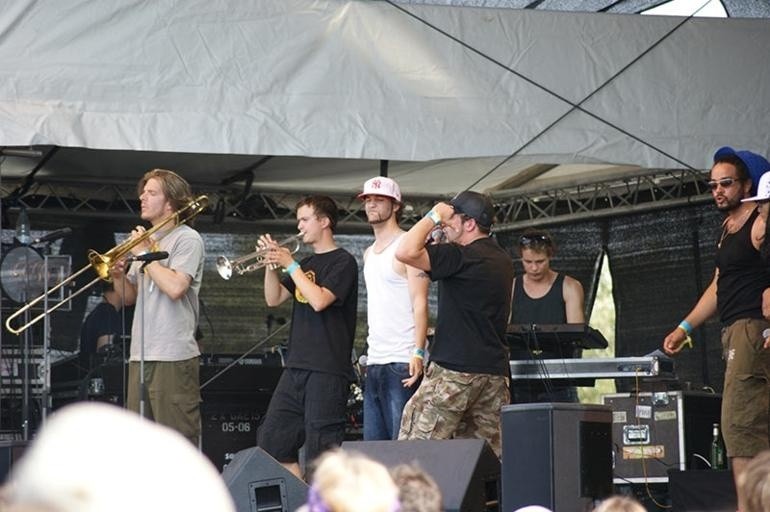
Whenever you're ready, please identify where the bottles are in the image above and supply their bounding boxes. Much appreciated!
[710,422,727,471]
[15,209,31,246]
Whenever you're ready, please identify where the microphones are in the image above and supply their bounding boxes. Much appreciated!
[425,229,442,245]
[359,355,367,366]
[31,227,72,245]
[126,251,169,261]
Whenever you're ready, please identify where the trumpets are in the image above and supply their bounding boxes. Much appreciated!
[216,232,305,280]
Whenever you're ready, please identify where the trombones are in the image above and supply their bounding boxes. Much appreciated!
[5,195,209,334]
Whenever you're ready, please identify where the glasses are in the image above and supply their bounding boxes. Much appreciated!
[520,235,552,245]
[707,178,740,191]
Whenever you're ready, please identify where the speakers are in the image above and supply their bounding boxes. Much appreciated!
[666,468,738,512]
[0,440,35,487]
[501,402,613,512]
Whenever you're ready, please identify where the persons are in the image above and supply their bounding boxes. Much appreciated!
[195,326,205,354]
[396,189,514,464]
[589,493,649,511]
[307,452,401,512]
[105,170,205,450]
[662,146,770,512]
[81,285,122,373]
[739,170,770,355]
[389,465,442,512]
[254,193,358,488]
[0,400,236,512]
[734,448,770,512]
[357,178,431,440]
[295,428,344,512]
[506,227,583,403]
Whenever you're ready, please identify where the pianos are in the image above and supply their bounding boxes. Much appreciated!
[510,355,678,378]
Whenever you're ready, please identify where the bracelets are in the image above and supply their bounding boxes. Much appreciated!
[414,348,424,358]
[761,327,770,338]
[426,210,440,226]
[282,261,300,274]
[679,321,693,336]
[136,249,157,256]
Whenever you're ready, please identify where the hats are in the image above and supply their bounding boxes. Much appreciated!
[713,146,770,194]
[357,175,402,204]
[740,171,770,203]
[444,189,495,229]
